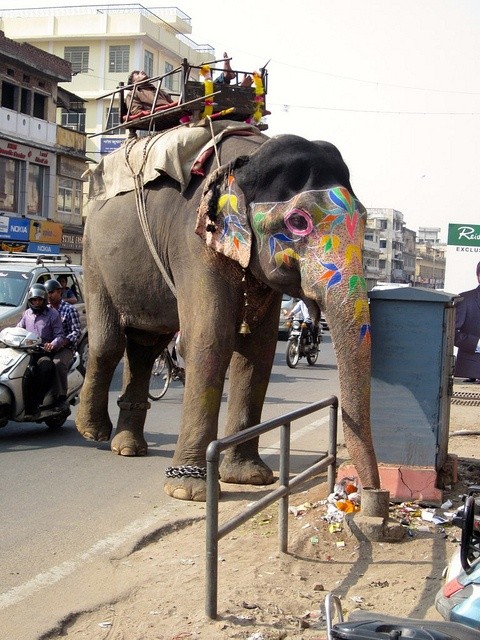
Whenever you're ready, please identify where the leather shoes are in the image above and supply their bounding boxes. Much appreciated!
[53,402,67,411]
[23,409,41,420]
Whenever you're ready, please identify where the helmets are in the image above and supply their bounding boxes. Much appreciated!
[44,279,62,294]
[26,283,48,312]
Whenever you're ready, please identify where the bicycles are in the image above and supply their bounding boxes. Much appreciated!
[149,346,187,400]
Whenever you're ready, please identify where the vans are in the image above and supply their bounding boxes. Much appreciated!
[279,294,301,340]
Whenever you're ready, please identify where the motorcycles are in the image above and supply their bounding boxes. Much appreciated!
[0,326,86,428]
[282,309,321,367]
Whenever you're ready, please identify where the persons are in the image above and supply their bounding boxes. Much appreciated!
[16,283,66,414]
[126,52,253,115]
[287,299,318,351]
[57,276,78,303]
[163,331,185,380]
[455,261,480,379]
[44,278,82,412]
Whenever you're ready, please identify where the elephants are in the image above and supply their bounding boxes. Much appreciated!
[74,131,381,503]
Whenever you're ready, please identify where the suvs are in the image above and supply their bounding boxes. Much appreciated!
[0,254,87,327]
